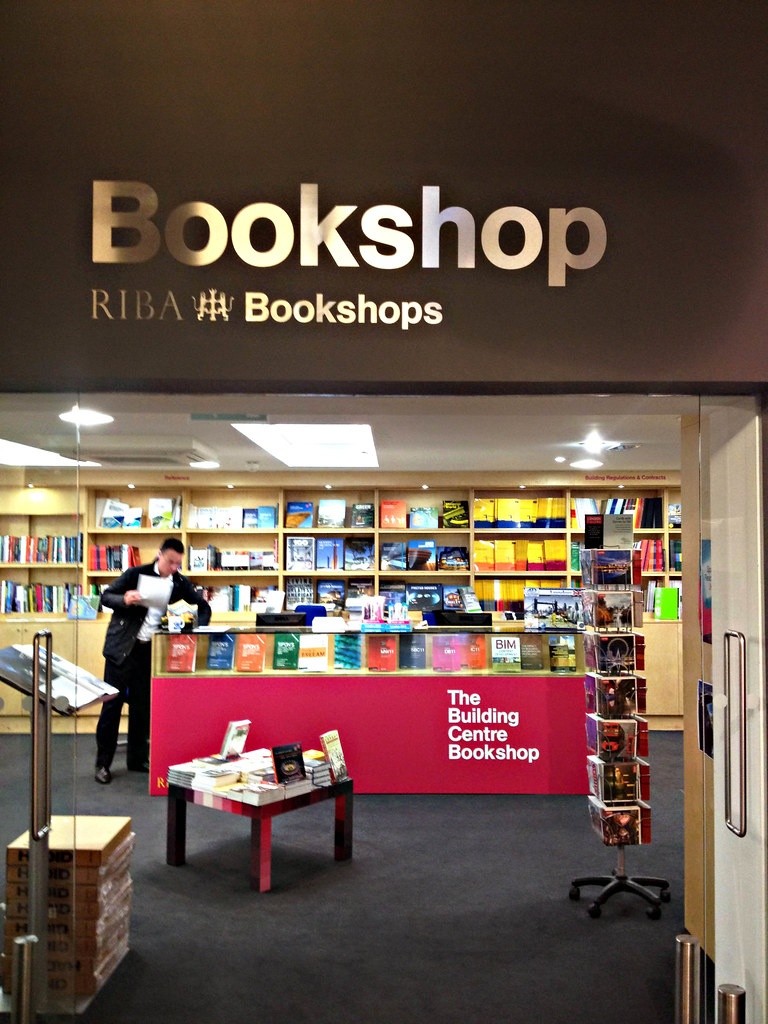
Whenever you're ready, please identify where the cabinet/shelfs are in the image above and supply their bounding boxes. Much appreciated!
[568,548,670,918]
[0,485,681,734]
[148,625,592,795]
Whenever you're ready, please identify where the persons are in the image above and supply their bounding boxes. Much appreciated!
[93,538,212,784]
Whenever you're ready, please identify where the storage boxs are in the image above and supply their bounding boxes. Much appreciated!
[1,815,136,998]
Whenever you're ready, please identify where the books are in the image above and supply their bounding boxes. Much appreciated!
[165,634,198,673]
[207,634,235,670]
[168,748,332,807]
[270,744,306,785]
[1,498,684,672]
[491,636,521,672]
[219,720,252,758]
[549,635,577,672]
[333,634,362,669]
[579,672,655,846]
[0,642,119,716]
[236,633,267,672]
[273,634,300,669]
[368,636,396,672]
[319,730,347,780]
[298,634,328,672]
[399,635,426,669]
[68,595,100,620]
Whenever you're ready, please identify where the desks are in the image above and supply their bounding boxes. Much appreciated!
[166,777,352,891]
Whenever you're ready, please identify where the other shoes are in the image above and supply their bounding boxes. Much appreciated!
[95,767,111,784]
[130,762,149,772]
